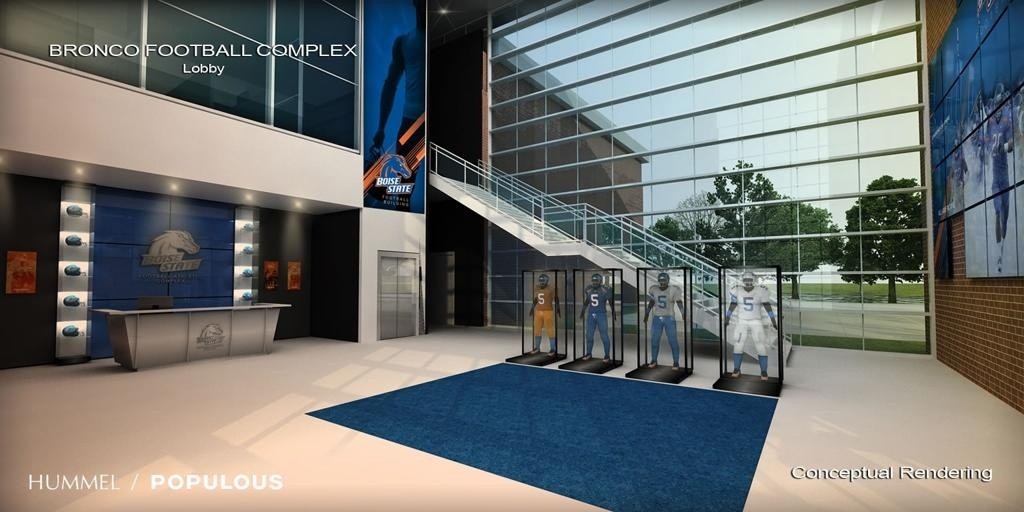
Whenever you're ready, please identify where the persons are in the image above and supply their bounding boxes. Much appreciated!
[580,274,617,363]
[643,273,685,372]
[951,82,1024,184]
[989,82,1015,273]
[725,271,777,382]
[529,275,562,357]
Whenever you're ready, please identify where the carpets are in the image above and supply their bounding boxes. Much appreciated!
[305,363,778,511]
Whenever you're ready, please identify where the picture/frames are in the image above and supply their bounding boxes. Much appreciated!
[5,250,39,294]
[263,260,302,294]
